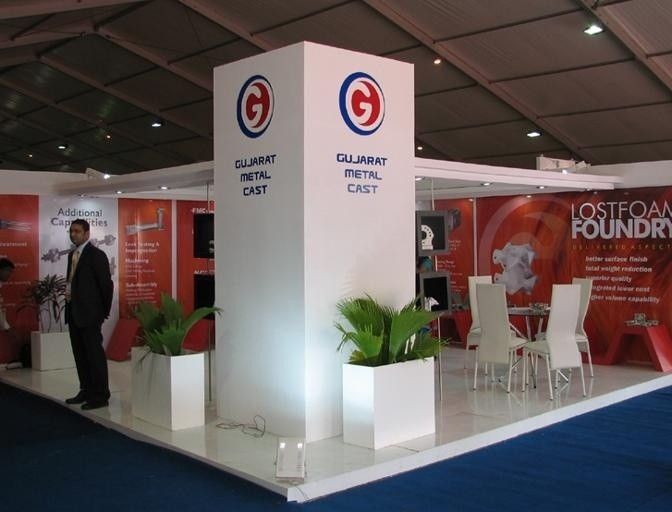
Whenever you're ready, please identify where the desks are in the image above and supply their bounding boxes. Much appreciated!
[605,323,672,374]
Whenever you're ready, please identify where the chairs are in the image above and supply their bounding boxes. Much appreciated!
[461,270,598,405]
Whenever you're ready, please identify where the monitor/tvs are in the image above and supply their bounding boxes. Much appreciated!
[416,272,452,316]
[416,211,449,257]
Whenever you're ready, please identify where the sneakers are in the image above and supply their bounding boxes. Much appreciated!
[66,390,86,403]
[81,399,108,409]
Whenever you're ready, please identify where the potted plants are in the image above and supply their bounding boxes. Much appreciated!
[119,288,226,432]
[334,294,453,452]
[17,272,91,373]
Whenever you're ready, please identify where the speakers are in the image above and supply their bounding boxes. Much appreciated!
[194,274,215,320]
[193,213,214,258]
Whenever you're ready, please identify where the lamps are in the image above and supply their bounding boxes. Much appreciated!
[582,9,605,36]
[275,434,310,485]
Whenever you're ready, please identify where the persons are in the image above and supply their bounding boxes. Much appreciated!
[64,219,114,409]
[414,255,432,273]
[0,258,14,332]
